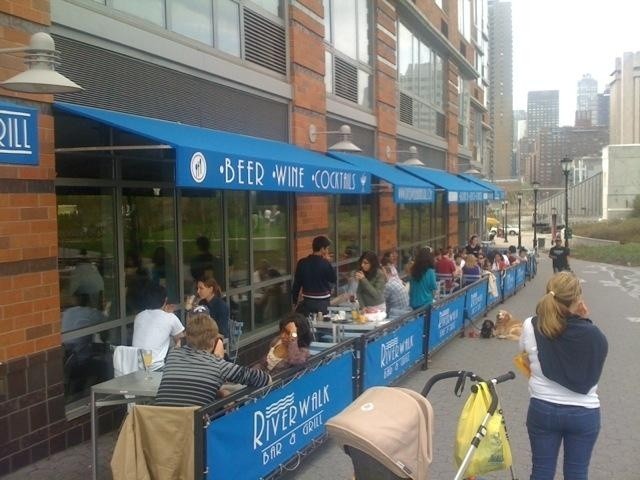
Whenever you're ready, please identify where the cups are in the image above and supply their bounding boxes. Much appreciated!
[358,310,366,323]
[352,308,359,319]
[339,311,345,320]
[323,316,331,322]
[186,296,195,309]
[142,349,153,380]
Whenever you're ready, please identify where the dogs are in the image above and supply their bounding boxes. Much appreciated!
[481,319,494,339]
[491,309,524,341]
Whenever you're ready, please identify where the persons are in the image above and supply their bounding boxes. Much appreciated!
[485,254,497,273]
[517,246,528,260]
[353,251,385,307]
[509,244,520,263]
[292,236,337,312]
[59,292,111,391]
[152,245,177,283]
[243,256,271,292]
[467,236,482,255]
[453,252,465,269]
[252,268,284,318]
[502,249,510,267]
[435,249,456,291]
[383,252,395,267]
[74,251,105,311]
[549,238,570,273]
[494,251,506,270]
[462,254,481,283]
[265,314,310,375]
[517,271,608,480]
[192,236,224,283]
[409,248,438,308]
[185,278,231,338]
[384,265,410,309]
[132,286,184,371]
[155,314,273,430]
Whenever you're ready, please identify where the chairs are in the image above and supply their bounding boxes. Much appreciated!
[110,345,151,372]
[313,325,340,343]
[322,307,364,340]
[229,319,243,363]
[309,341,338,357]
[461,274,480,288]
[309,322,338,343]
[240,301,262,325]
[90,343,113,382]
[436,274,453,285]
[66,356,94,392]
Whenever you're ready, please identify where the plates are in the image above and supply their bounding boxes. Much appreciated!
[333,320,352,322]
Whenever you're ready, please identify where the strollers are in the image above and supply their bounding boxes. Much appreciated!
[325,371,516,480]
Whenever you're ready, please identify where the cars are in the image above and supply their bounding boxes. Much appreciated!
[490,223,519,235]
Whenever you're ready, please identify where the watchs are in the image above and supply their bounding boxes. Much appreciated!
[289,332,298,339]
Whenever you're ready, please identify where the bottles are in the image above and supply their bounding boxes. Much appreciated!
[352,307,356,323]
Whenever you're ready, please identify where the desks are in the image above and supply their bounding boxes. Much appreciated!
[91,371,247,480]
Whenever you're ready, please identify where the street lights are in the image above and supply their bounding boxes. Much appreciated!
[502,200,505,237]
[505,200,508,243]
[559,157,574,244]
[516,193,523,249]
[533,182,539,256]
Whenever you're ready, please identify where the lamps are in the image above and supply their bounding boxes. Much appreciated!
[385,146,424,166]
[308,124,361,152]
[0,31,85,94]
[456,163,481,174]
